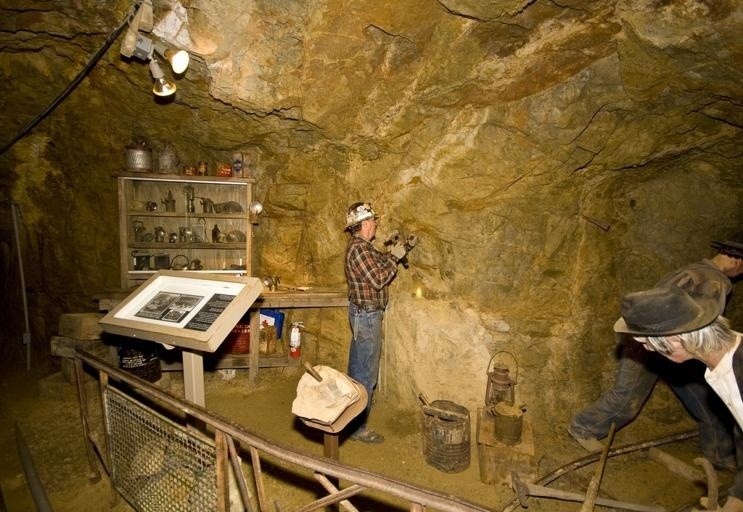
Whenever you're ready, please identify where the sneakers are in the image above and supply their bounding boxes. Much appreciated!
[345,429,385,444]
[566,424,605,454]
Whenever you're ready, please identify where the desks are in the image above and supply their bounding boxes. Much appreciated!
[99,284,351,389]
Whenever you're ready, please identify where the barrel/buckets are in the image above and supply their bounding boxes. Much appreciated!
[230,323,250,355]
[421,401,470,473]
[119,341,161,383]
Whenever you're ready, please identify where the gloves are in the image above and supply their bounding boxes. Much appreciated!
[407,233,417,247]
[390,239,407,259]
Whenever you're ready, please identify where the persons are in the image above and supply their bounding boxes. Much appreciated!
[564,233,741,472]
[610,282,742,457]
[342,200,418,446]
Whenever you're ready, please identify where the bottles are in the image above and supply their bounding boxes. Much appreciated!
[198,160,208,176]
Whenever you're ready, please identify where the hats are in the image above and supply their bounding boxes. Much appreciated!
[611,283,723,338]
[708,227,743,254]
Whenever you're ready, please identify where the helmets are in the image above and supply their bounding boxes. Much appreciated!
[343,201,380,232]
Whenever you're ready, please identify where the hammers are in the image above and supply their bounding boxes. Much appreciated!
[511,471,667,512]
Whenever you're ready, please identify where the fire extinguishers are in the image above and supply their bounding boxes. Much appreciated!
[289,322,306,357]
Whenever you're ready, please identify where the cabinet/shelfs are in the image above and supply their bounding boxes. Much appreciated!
[110,170,256,291]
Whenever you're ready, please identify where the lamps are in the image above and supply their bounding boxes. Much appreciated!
[484,352,520,416]
[0,0,191,152]
